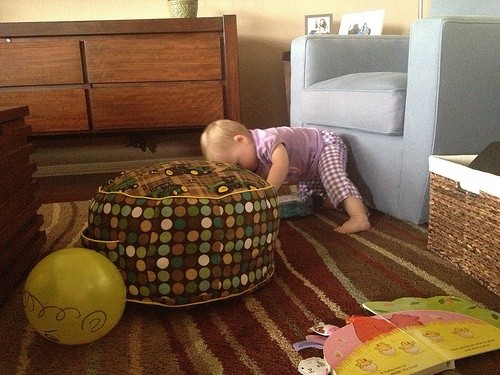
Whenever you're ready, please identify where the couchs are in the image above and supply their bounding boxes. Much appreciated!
[289,0,500,225]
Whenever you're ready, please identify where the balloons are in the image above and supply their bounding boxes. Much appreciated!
[22,248,127,346]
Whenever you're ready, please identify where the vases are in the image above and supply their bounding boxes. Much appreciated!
[167,0,198,18]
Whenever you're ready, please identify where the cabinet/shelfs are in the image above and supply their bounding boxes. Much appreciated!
[0,15,243,178]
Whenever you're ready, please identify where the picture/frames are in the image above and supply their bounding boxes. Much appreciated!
[338,9,386,35]
[305,13,333,35]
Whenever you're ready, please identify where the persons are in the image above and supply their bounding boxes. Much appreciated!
[348,22,371,36]
[199,119,371,235]
[318,19,328,32]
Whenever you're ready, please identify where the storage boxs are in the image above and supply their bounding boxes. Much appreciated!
[428,154,500,296]
